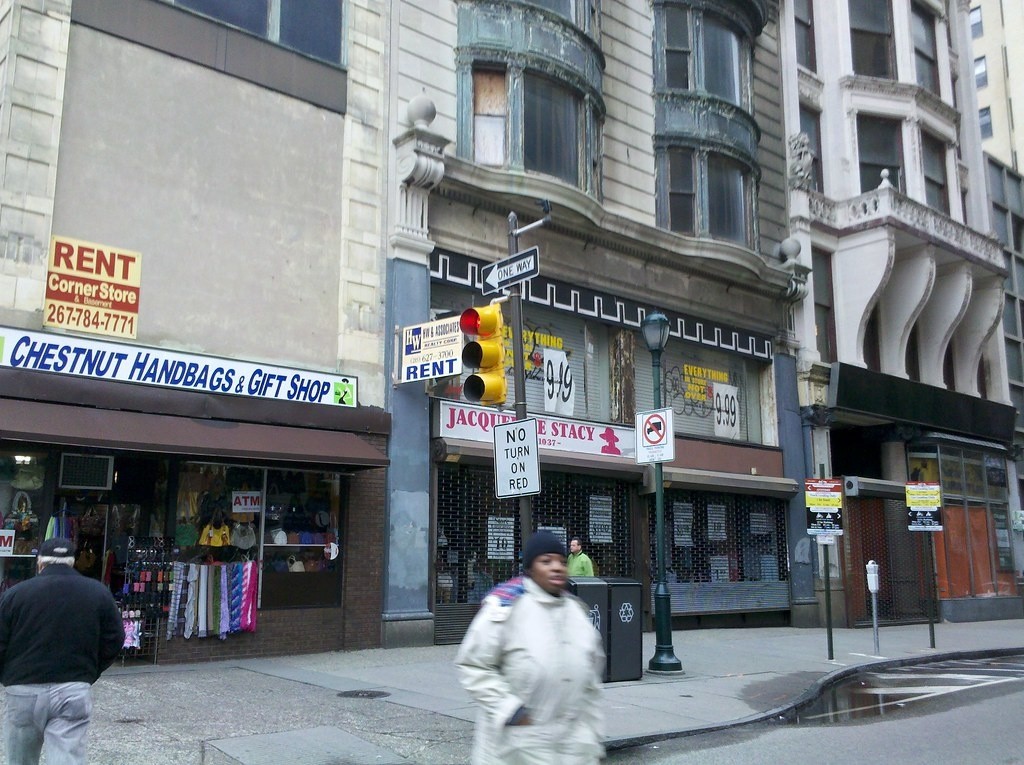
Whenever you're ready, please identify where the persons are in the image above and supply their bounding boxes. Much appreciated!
[454,530,606,764]
[0,539,125,764]
[565,537,595,578]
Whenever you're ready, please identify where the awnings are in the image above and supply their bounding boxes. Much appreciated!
[1,396,391,467]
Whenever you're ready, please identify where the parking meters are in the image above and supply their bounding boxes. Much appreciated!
[865,559,881,658]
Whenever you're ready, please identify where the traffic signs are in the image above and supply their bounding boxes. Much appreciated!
[481,244,542,296]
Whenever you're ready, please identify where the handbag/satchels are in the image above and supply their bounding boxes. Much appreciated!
[263,550,341,576]
[269,528,336,544]
[75,540,96,573]
[266,469,307,533]
[306,473,332,513]
[0,569,30,595]
[80,505,105,536]
[174,465,260,566]
[0,461,45,555]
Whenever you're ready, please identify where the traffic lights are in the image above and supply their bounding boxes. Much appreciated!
[457,304,510,406]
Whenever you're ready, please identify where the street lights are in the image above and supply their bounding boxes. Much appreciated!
[641,307,684,676]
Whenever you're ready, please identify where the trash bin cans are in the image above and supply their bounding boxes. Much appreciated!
[565,576,645,685]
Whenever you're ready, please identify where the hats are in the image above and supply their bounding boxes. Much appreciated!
[523,532,569,569]
[315,511,330,527]
[40,538,77,556]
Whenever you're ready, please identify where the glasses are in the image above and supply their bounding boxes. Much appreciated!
[127,536,179,572]
